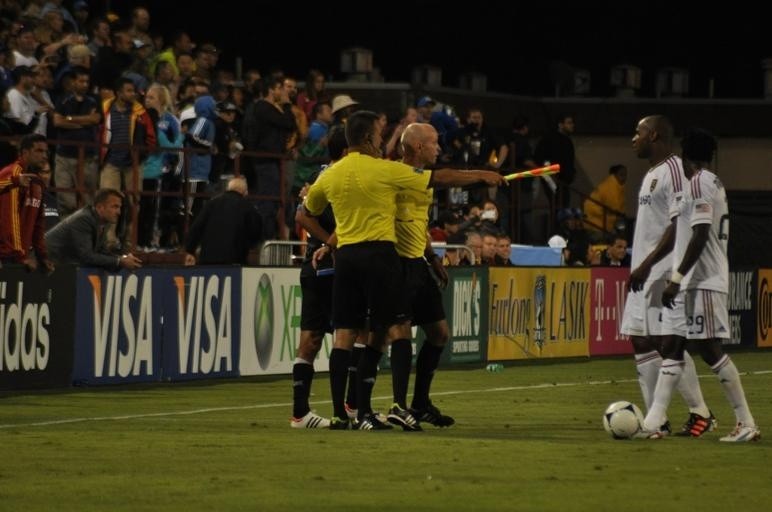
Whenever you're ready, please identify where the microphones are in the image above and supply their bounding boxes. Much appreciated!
[367,135,381,154]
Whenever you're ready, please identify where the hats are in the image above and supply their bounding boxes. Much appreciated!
[11,65,33,75]
[72,44,95,58]
[417,96,434,107]
[331,96,358,113]
[132,40,149,49]
[556,207,587,222]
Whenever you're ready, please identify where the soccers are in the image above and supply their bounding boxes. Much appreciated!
[603,402,643,439]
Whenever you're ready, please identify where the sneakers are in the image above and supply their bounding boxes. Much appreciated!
[289,401,455,432]
[633,407,761,443]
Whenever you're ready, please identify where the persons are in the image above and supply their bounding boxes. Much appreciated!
[1,0,361,267]
[375,94,628,267]
[312,122,455,427]
[291,126,368,428]
[636,129,760,442]
[623,117,718,441]
[299,111,508,432]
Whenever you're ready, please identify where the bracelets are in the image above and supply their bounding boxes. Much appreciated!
[671,271,683,283]
